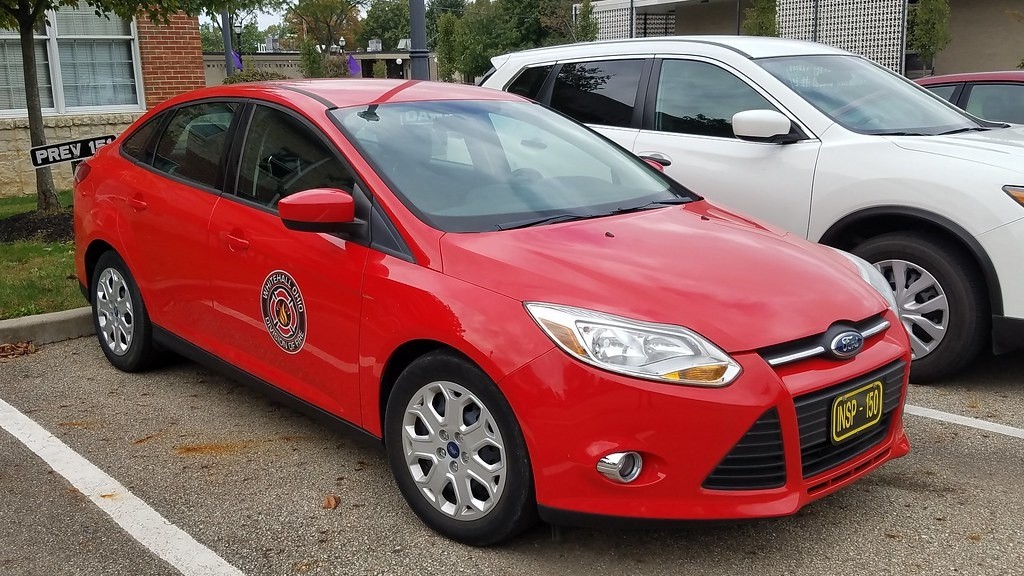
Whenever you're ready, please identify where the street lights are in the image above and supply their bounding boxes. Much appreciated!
[233,19,243,72]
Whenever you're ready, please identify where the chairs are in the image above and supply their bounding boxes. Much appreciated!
[171,123,228,184]
[244,111,355,205]
[378,124,453,211]
[676,64,734,134]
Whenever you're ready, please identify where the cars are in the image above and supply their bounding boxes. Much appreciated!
[73,78,911,549]
[828,70,1024,137]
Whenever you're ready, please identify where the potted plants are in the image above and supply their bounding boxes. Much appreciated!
[906,0,951,75]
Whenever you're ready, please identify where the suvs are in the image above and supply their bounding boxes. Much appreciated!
[446,37,1024,385]
